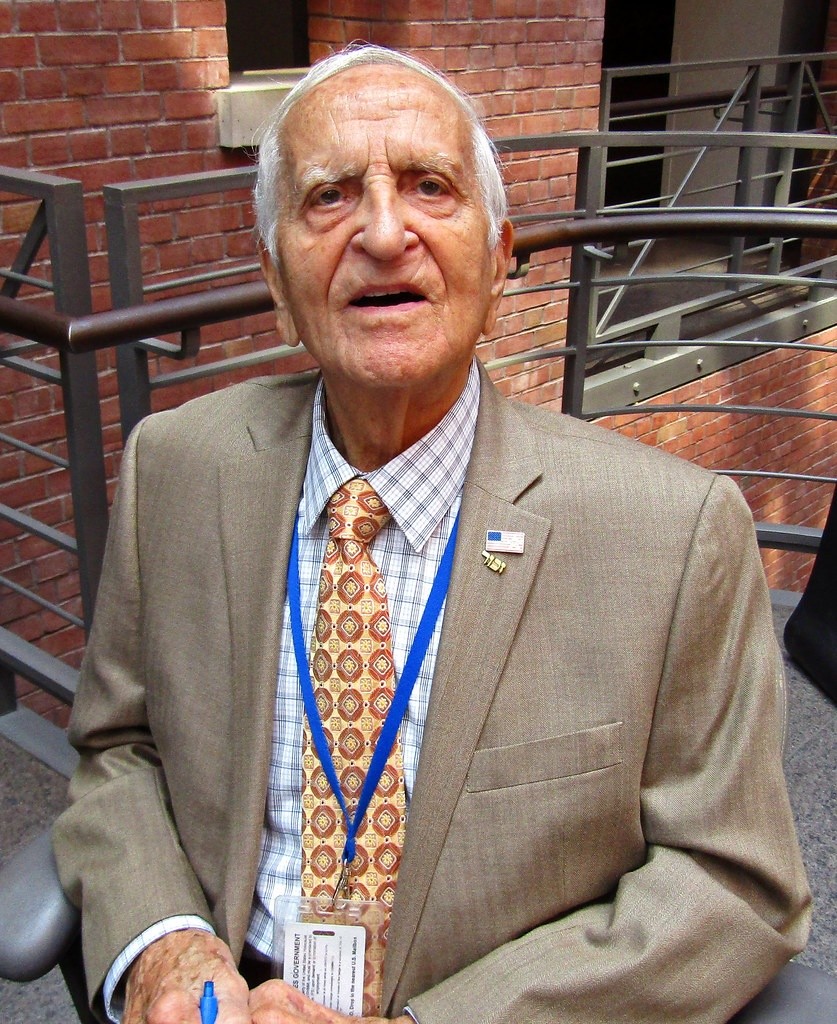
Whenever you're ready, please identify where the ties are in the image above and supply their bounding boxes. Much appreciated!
[295,479,407,1019]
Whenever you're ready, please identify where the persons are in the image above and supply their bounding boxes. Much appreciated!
[49,38,813,1024]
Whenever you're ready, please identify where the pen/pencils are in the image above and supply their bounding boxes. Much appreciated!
[201,981,219,1024]
